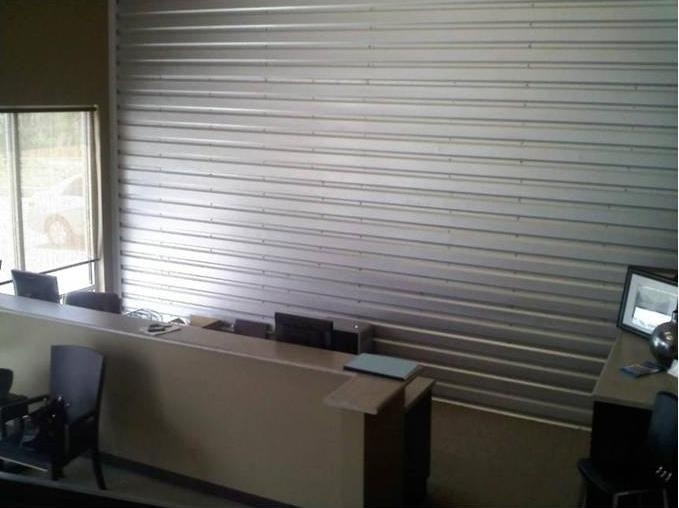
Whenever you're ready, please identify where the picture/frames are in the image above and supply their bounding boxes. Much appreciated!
[617,264,678,341]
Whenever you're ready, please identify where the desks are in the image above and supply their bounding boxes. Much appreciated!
[589,327,677,461]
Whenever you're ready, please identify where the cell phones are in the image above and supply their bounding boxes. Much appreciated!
[149,327,166,332]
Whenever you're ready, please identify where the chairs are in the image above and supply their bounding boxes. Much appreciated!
[1,342,106,490]
[578,391,677,506]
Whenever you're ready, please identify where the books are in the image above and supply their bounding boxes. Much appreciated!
[343,351,424,380]
[617,359,664,380]
[138,321,182,338]
